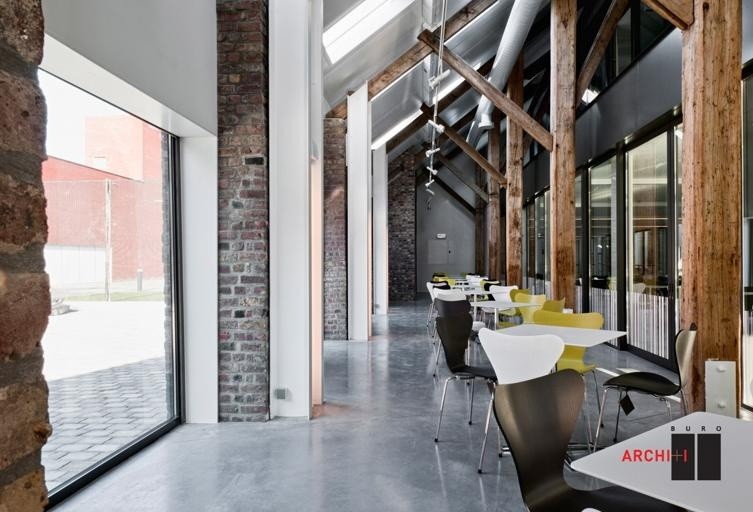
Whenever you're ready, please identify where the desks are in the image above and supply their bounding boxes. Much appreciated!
[569,409,752,512]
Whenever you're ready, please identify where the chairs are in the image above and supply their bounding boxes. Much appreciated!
[424,270,700,512]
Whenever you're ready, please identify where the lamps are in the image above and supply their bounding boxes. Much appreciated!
[422,69,452,188]
